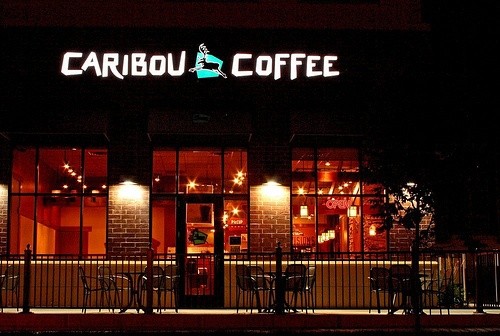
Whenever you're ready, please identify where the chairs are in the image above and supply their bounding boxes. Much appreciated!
[0,264,22,308]
[80,264,181,314]
[368,264,453,316]
[235,263,315,313]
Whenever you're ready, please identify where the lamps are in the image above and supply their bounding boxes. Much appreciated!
[347,202,357,218]
[300,205,309,218]
[48,170,63,198]
[318,228,335,244]
